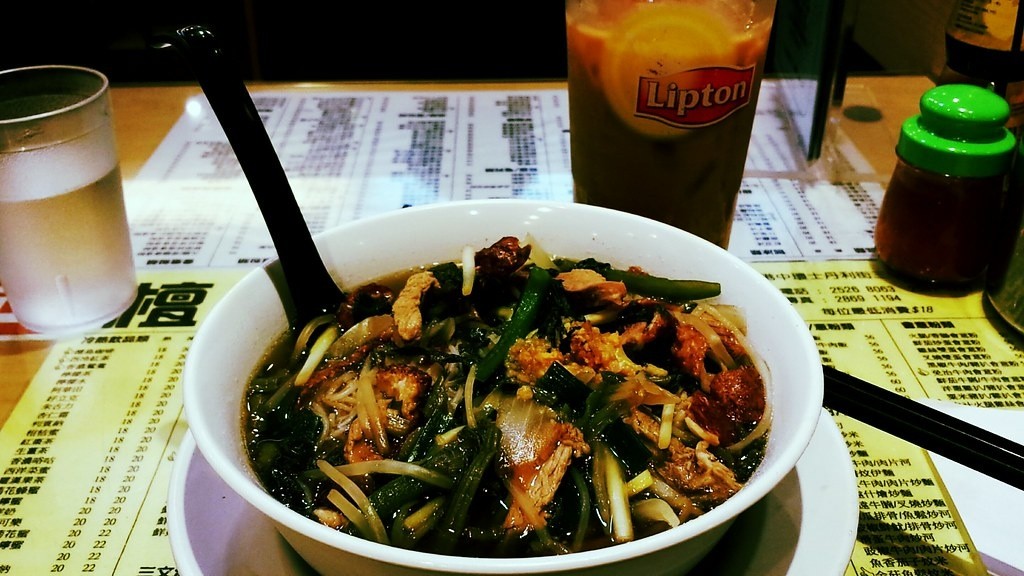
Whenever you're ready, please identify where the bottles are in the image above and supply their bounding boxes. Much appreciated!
[875,83,1017,290]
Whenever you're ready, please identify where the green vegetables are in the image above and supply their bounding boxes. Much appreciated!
[244,252,764,563]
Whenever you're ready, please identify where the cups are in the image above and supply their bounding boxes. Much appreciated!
[1,66,141,333]
[564,0,776,258]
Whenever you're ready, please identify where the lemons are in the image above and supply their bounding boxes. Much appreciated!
[603,15,725,140]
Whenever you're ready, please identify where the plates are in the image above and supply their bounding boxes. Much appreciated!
[167,380,858,576]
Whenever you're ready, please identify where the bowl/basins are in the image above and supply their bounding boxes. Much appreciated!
[183,199,826,575]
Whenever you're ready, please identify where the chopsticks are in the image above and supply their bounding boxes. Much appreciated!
[808,358,1024,498]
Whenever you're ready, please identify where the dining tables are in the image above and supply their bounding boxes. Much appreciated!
[0,65,1024,576]
